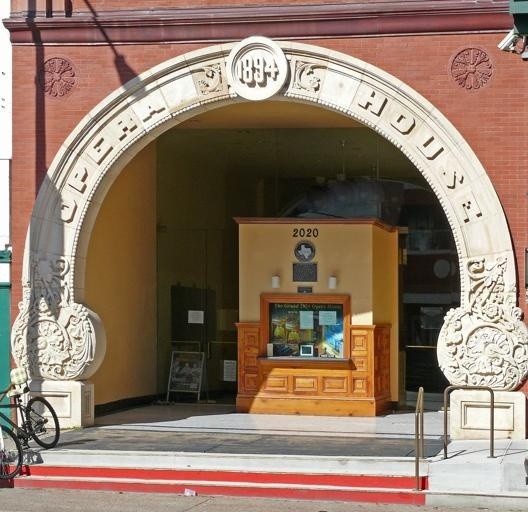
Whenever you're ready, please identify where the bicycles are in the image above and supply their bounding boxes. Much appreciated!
[0,383,60,480]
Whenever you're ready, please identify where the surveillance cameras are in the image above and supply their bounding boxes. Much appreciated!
[497,29,528,56]
